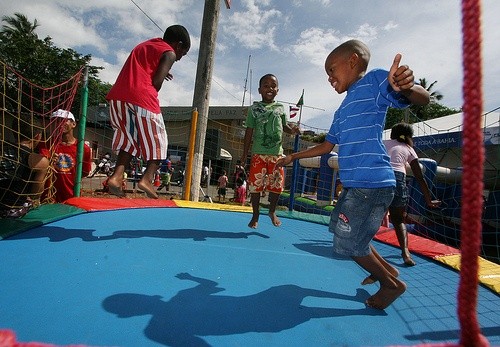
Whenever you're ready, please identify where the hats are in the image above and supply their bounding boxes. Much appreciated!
[50,109,75,122]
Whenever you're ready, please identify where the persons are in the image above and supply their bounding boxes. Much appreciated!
[130,154,248,207]
[273,39,430,310]
[239,74,303,230]
[105,25,191,199]
[0,109,91,218]
[382,122,448,266]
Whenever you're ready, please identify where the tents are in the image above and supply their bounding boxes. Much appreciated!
[383,110,500,149]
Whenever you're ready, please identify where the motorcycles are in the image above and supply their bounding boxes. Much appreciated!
[88,159,116,178]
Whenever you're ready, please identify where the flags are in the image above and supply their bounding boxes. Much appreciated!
[290,91,305,118]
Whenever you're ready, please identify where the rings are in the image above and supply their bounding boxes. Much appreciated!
[276,163,279,165]
